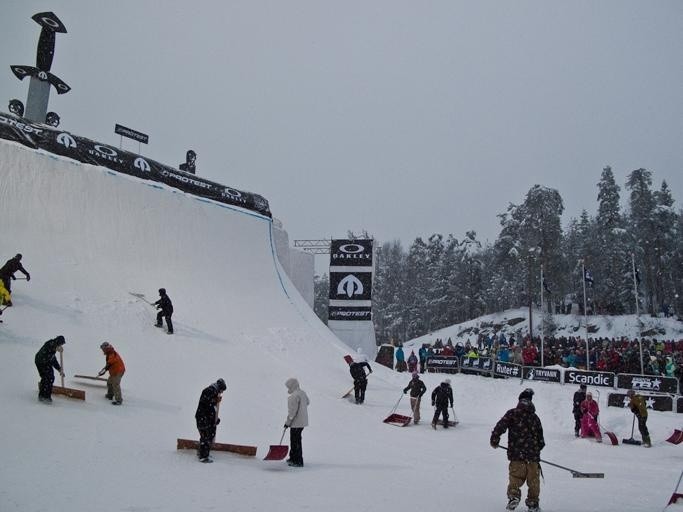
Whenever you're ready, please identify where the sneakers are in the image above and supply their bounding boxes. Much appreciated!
[527,501,539,511]
[197,454,303,467]
[506,497,520,510]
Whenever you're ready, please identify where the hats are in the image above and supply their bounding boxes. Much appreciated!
[217,379,226,390]
[519,389,534,400]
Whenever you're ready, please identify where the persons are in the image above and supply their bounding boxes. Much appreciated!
[430,378,455,431]
[99,340,126,406]
[150,287,175,335]
[570,381,589,438]
[667,304,674,318]
[401,370,427,425]
[578,390,602,443]
[489,387,545,512]
[0,252,31,308]
[194,377,227,464]
[545,293,594,316]
[34,335,67,403]
[624,388,651,448]
[343,354,373,405]
[393,328,683,395]
[283,377,310,468]
[661,301,668,319]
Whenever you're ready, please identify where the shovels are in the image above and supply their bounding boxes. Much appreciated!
[587,411,618,445]
[263,427,288,460]
[665,429,683,445]
[383,391,421,427]
[662,471,683,512]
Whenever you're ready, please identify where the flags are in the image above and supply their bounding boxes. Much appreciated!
[634,262,641,287]
[543,273,555,294]
[584,266,594,288]
